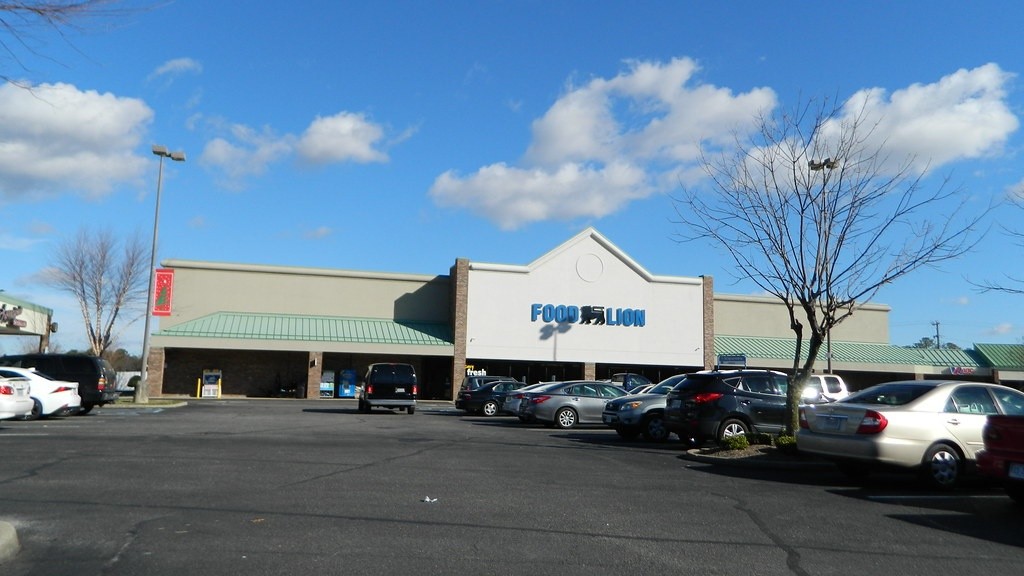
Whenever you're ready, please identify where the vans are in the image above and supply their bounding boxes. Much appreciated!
[461,376,519,391]
[0,353,120,416]
[359,363,418,415]
[798,373,850,403]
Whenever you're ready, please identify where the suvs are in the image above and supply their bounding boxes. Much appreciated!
[663,368,839,447]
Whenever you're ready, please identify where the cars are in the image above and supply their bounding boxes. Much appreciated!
[455,381,529,416]
[975,411,1024,522]
[628,384,674,395]
[0,369,35,421]
[520,380,633,429]
[1,367,81,421]
[503,381,565,423]
[796,379,1024,494]
[602,374,690,445]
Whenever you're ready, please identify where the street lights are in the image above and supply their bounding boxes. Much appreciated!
[134,145,189,406]
